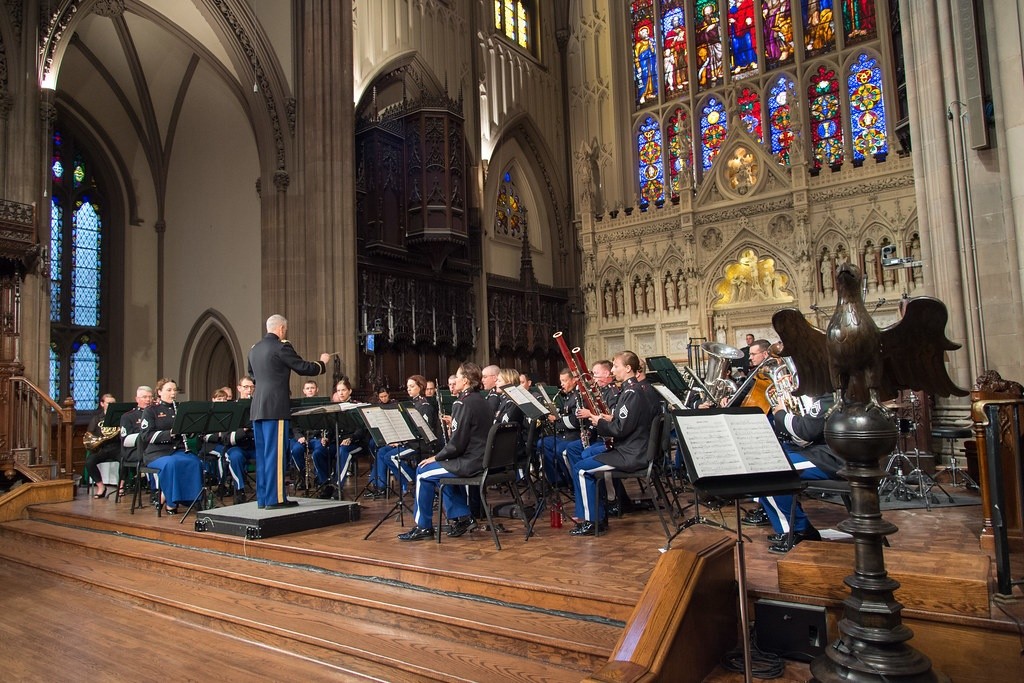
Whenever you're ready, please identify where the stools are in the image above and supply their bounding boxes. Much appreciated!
[932,426,977,489]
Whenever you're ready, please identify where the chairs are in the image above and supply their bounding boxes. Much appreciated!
[85,400,850,551]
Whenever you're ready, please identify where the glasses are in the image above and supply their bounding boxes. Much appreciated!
[241,384,255,389]
[748,350,764,356]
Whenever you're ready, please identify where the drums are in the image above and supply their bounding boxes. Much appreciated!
[896,417,913,436]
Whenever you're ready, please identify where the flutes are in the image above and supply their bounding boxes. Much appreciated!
[571,381,598,449]
[434,377,452,445]
[171,397,192,455]
[485,386,496,399]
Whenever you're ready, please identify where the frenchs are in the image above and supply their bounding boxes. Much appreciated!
[82,419,122,452]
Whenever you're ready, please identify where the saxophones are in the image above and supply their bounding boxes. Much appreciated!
[535,386,564,437]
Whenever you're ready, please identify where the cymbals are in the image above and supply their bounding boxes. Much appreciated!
[886,403,904,408]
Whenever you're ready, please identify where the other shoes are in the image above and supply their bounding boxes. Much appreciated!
[233,489,247,505]
[150,489,163,510]
[315,484,345,501]
[119,490,125,497]
[94,485,107,499]
[296,481,311,491]
[165,504,178,515]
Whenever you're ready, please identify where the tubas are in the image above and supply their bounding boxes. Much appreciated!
[763,341,823,448]
[699,341,746,408]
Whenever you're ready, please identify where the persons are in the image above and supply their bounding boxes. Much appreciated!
[707,230,716,241]
[646,281,654,310]
[835,252,848,265]
[207,311,367,509]
[538,350,663,535]
[799,256,815,281]
[779,216,786,232]
[665,276,676,306]
[605,287,613,315]
[735,158,750,182]
[584,287,595,312]
[634,282,644,310]
[677,275,687,305]
[736,334,830,552]
[864,246,877,284]
[369,361,542,542]
[821,256,833,289]
[881,254,894,281]
[615,285,624,313]
[84,376,203,513]
[686,272,697,301]
[911,239,923,278]
[731,258,786,301]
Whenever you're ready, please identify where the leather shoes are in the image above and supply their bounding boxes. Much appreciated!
[397,525,435,542]
[217,484,234,497]
[569,520,609,537]
[740,507,771,526]
[767,525,824,554]
[258,500,299,510]
[363,488,386,499]
[446,514,477,537]
